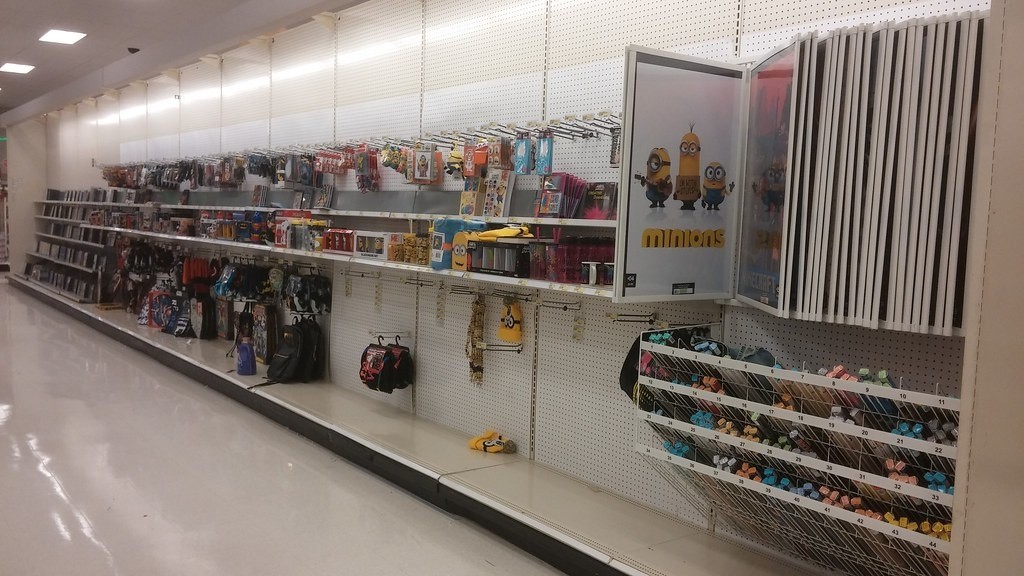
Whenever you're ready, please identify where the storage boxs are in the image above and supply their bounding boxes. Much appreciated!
[94,126,620,366]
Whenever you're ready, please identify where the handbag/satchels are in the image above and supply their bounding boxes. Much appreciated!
[107,239,329,341]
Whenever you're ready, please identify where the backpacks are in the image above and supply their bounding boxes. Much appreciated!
[376,344,412,394]
[359,344,395,389]
[290,315,326,383]
[267,316,302,385]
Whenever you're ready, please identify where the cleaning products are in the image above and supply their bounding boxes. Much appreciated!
[237,336,258,376]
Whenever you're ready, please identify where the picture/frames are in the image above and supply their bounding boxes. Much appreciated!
[611,8,990,338]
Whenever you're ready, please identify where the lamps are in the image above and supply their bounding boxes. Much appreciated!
[249,35,274,49]
[130,79,148,91]
[82,97,97,108]
[103,88,119,101]
[198,54,224,70]
[311,12,340,30]
[162,67,180,81]
[64,104,78,113]
[48,110,60,119]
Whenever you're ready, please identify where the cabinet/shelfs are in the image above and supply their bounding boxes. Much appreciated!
[14,200,116,305]
[631,319,962,576]
[80,201,617,300]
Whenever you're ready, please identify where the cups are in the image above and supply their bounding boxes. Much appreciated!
[529,237,615,285]
